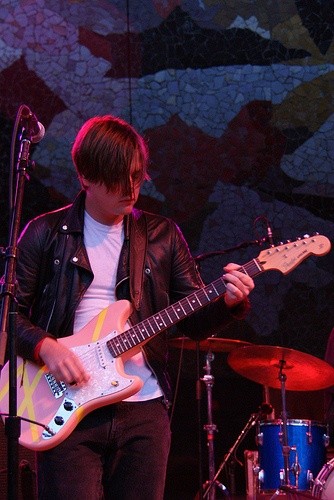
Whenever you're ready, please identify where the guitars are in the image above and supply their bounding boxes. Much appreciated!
[0,232,331,451]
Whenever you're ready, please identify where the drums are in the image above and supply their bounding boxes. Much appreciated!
[255,418,330,500]
[311,457,334,500]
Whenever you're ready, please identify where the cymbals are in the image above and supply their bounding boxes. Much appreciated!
[227,345,334,391]
[166,337,253,351]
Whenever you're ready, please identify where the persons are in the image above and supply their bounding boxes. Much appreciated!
[1,115,254,500]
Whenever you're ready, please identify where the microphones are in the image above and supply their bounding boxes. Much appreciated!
[21,106,45,143]
[267,227,274,247]
